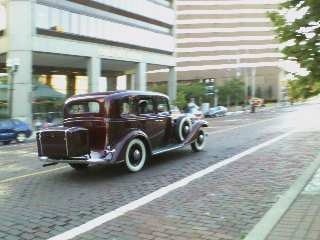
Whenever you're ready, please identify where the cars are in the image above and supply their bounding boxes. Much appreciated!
[0,119,33,147]
[205,106,228,118]
[36,90,209,173]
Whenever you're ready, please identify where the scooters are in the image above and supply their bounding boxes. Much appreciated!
[191,106,204,121]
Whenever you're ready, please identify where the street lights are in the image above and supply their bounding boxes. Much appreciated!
[5,57,21,119]
